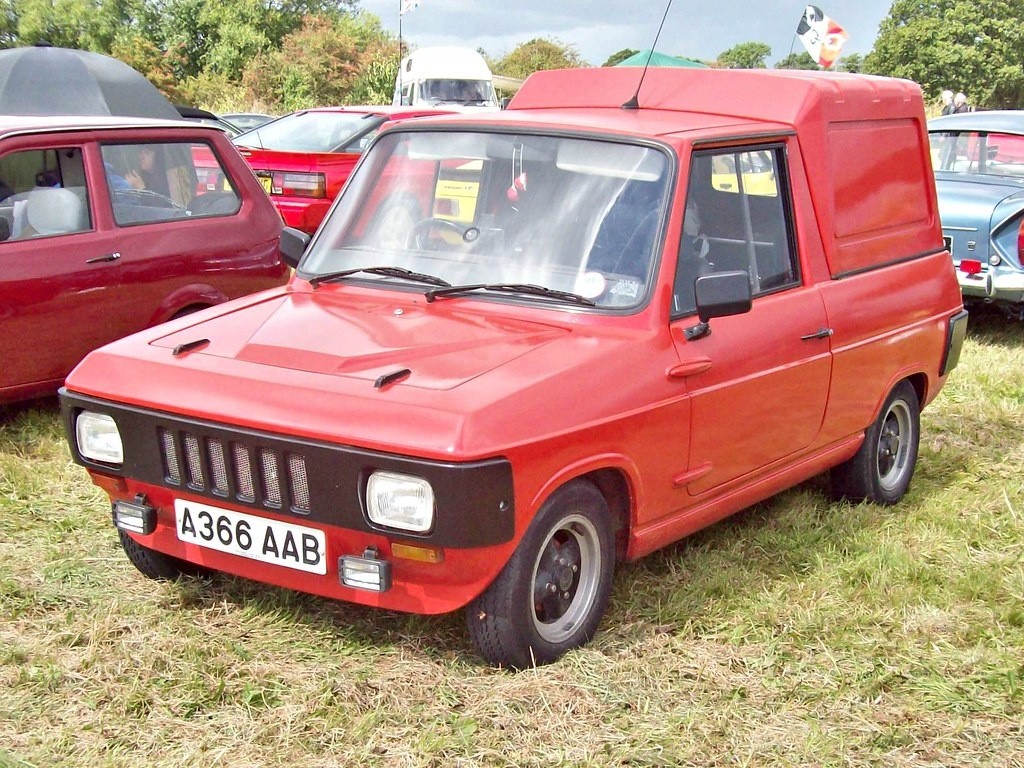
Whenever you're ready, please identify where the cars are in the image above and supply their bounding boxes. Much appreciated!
[171,42,1023,319]
[0,112,295,415]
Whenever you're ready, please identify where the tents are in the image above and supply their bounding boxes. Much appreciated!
[0,38,199,208]
[613,49,710,68]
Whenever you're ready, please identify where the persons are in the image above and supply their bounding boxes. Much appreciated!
[459,84,476,100]
[937,90,970,161]
[68,144,170,198]
[39,170,61,188]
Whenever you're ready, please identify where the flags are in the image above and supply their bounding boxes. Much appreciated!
[795,5,849,69]
[399,0,422,17]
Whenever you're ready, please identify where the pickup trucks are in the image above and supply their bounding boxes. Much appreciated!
[57,69,969,676]
